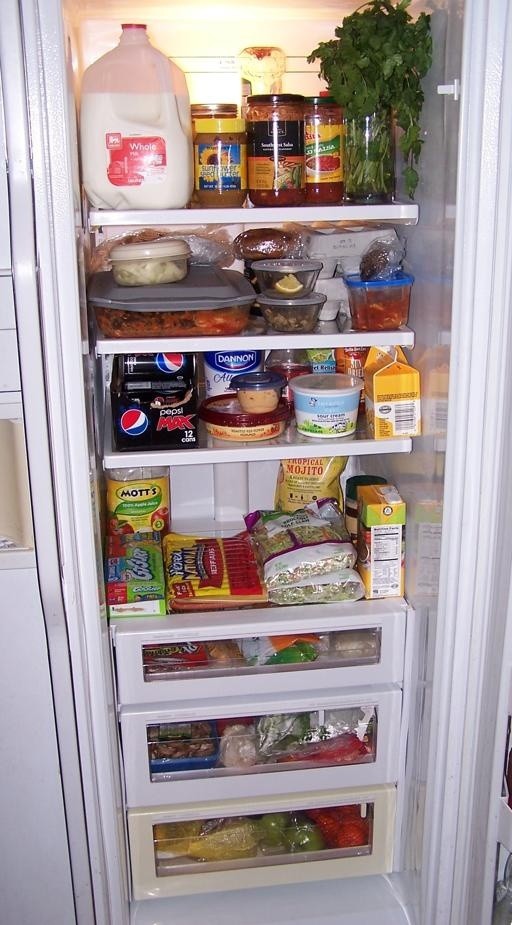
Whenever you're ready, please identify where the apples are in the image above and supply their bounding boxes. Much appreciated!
[106,518,134,537]
[261,812,325,852]
[151,507,169,531]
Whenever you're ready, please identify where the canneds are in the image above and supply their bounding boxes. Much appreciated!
[115,352,196,406]
[203,350,265,401]
[190,91,349,207]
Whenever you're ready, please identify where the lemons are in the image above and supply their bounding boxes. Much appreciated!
[275,274,303,295]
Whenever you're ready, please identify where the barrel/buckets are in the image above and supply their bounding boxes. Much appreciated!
[81,24,194,210]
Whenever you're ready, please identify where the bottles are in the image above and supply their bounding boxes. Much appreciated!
[264,350,312,430]
[344,475,387,544]
